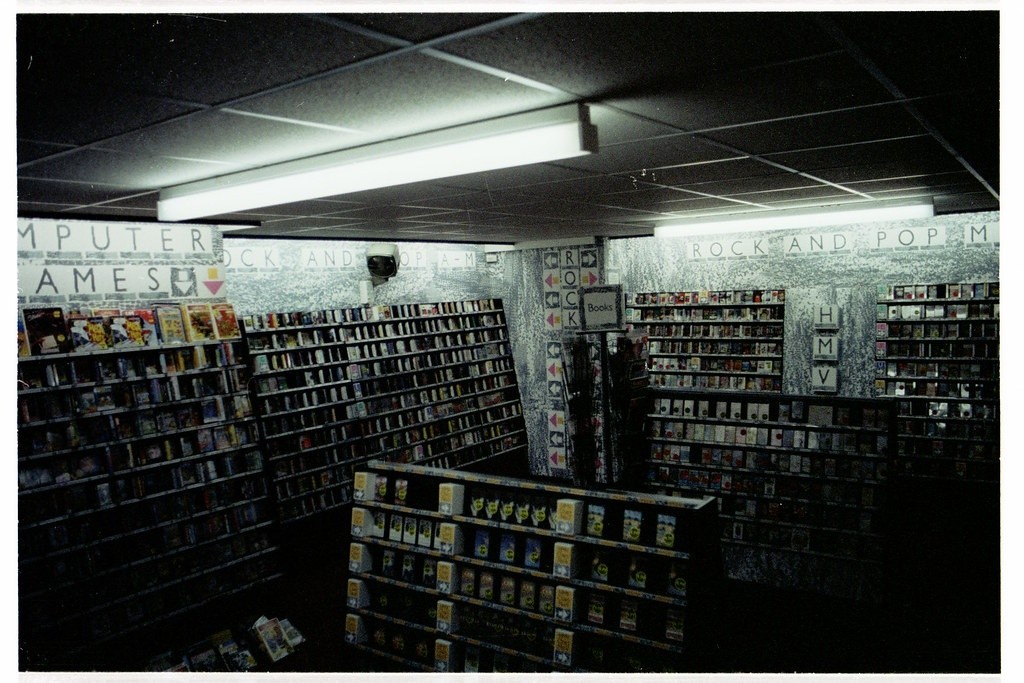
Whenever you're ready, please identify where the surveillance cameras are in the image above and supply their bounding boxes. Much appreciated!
[365,244,399,279]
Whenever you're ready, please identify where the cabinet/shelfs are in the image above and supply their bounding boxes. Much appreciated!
[873,282,1000,485]
[641,387,900,646]
[18,321,286,670]
[235,296,531,606]
[343,459,720,673]
[623,289,786,393]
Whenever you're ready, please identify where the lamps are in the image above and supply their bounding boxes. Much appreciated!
[155,100,600,221]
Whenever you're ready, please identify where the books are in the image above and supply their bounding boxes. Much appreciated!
[18,305,304,672]
